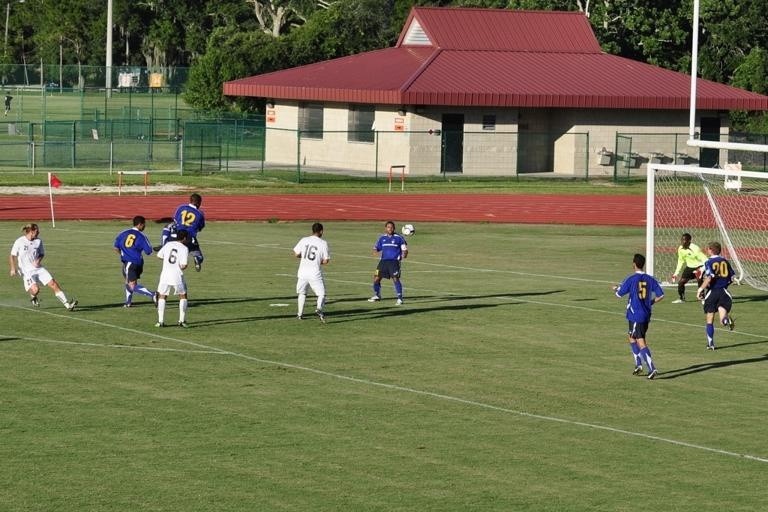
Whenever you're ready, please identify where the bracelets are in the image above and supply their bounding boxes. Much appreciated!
[698,288,703,293]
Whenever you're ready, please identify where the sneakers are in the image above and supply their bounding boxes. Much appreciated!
[193,255,201,272]
[152,245,163,252]
[646,369,658,379]
[633,364,643,375]
[178,320,189,327]
[66,300,78,311]
[395,299,403,306]
[122,302,131,308]
[727,314,735,330]
[671,298,686,304]
[155,322,164,327]
[297,314,302,320]
[367,294,380,302]
[705,344,715,350]
[315,309,324,321]
[30,297,40,308]
[151,291,158,308]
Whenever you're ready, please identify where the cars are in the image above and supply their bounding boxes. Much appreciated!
[45,80,72,92]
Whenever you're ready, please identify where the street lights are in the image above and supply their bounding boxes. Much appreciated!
[4,0,25,56]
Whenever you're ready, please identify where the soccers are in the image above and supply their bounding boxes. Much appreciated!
[401,224,414,236]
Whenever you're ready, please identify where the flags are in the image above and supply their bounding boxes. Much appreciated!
[51,175,61,188]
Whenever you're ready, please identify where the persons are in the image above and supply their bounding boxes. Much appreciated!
[5,91,13,116]
[113,216,159,308]
[154,230,189,328]
[293,223,330,324]
[613,254,665,379]
[671,234,709,305]
[10,223,78,311]
[153,194,205,272]
[696,242,736,351]
[368,222,408,305]
